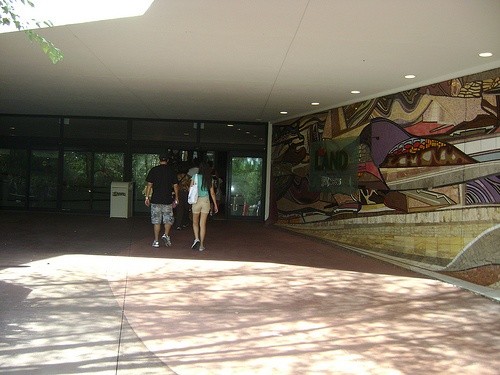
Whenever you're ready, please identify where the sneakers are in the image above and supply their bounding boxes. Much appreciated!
[199,247,206,251]
[161,234,171,247]
[152,240,159,248]
[191,240,200,249]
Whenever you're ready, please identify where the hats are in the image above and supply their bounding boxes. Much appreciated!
[159,155,167,160]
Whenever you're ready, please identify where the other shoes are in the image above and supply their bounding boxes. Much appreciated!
[177,227,181,230]
[183,224,189,227]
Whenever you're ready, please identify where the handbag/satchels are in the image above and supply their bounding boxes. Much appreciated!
[188,173,198,204]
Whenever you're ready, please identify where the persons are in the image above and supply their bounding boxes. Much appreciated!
[145,153,179,247]
[187,161,218,251]
[202,155,226,217]
[176,164,190,230]
[187,159,202,225]
